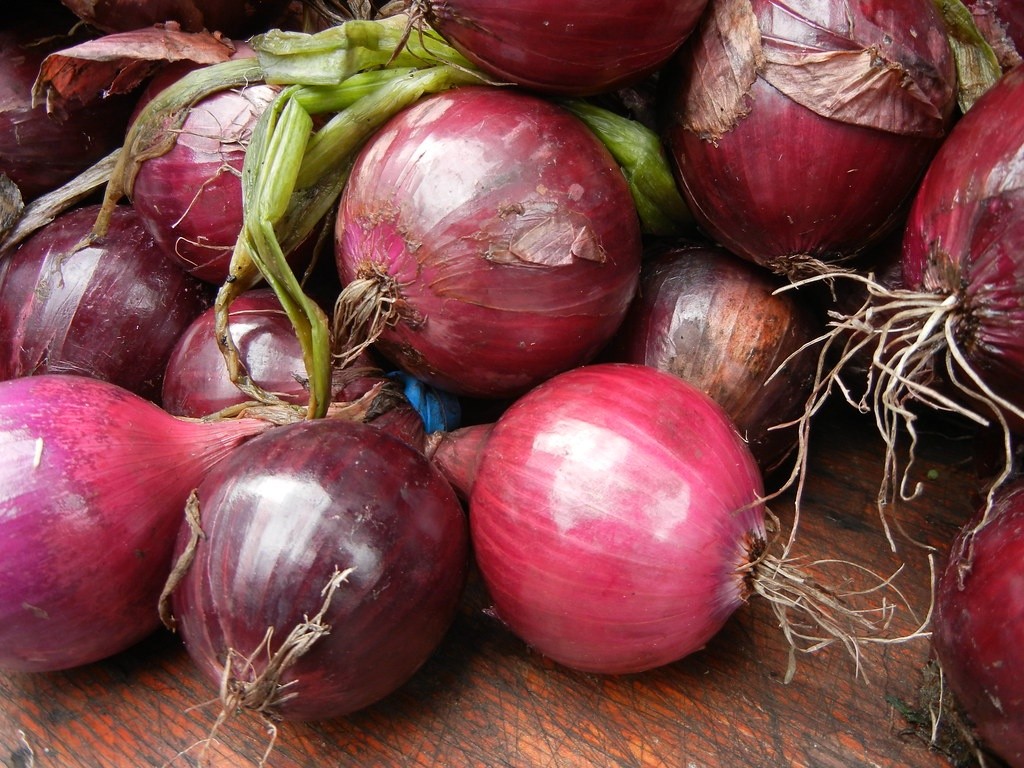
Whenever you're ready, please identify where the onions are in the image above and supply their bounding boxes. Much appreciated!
[0,0,1024,767]
